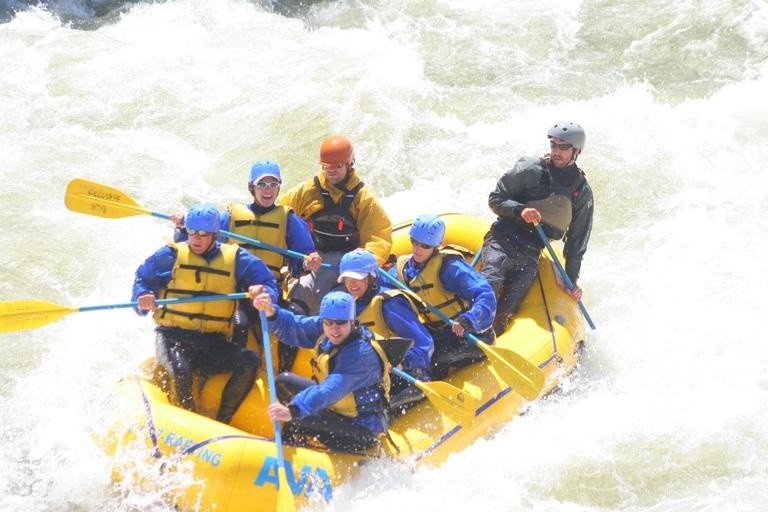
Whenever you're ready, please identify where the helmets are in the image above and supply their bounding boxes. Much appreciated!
[548,120,586,151]
[184,203,221,234]
[409,213,446,247]
[319,136,355,167]
[248,158,282,185]
[337,247,378,284]
[319,291,355,322]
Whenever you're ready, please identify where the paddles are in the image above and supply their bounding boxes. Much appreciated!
[0,286,268,334]
[64,178,333,271]
[378,266,546,402]
[259,306,298,512]
[390,368,479,434]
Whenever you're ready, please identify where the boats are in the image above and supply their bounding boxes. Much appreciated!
[92,211,584,511]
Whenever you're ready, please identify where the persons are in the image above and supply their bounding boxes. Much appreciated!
[277,133,393,315]
[337,248,436,415]
[379,214,498,378]
[479,119,597,337]
[251,291,394,454]
[169,159,322,347]
[129,202,283,424]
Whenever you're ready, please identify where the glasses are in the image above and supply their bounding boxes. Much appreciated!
[254,181,279,189]
[323,319,347,326]
[410,236,432,249]
[187,229,209,237]
